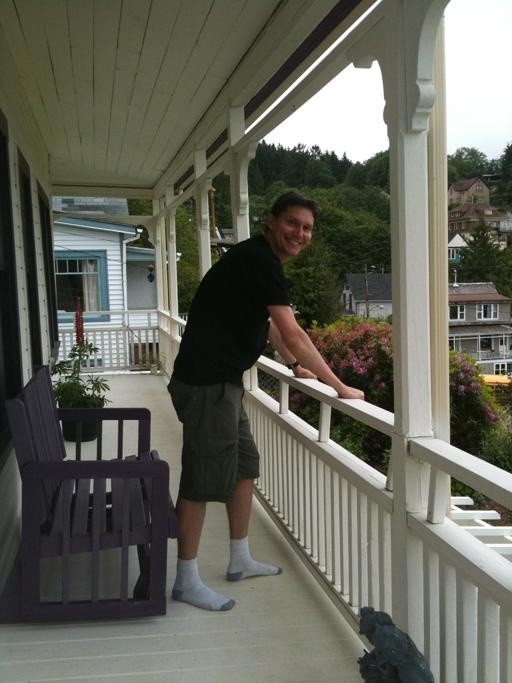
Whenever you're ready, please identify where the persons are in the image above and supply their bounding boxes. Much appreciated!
[158,189,367,611]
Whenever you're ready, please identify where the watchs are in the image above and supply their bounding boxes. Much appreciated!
[286,360,300,370]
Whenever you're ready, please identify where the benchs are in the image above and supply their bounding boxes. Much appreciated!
[0,364,178,624]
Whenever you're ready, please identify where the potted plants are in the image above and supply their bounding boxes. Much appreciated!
[48,338,112,441]
